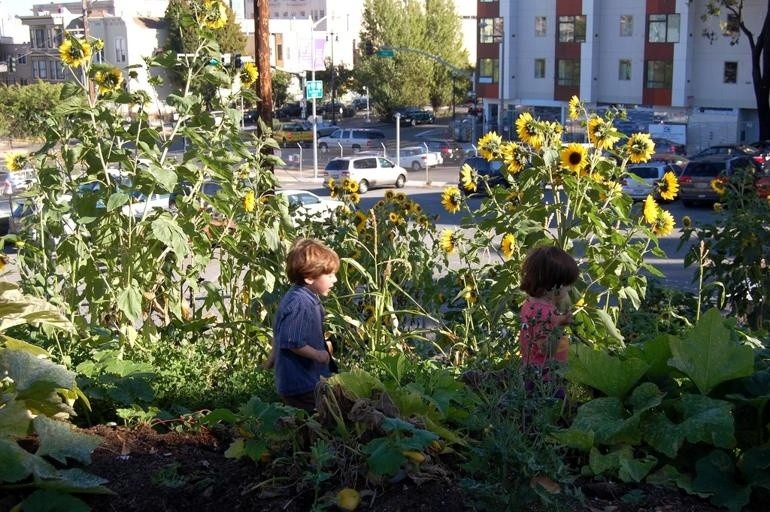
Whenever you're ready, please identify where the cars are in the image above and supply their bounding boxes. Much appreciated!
[172,108,258,126]
[0,159,236,258]
[275,190,351,230]
[272,98,511,196]
[622,138,770,208]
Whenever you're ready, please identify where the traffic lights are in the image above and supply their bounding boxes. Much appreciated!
[235,53,242,68]
[365,40,373,55]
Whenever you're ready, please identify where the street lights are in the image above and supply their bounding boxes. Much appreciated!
[363,85,370,118]
[309,15,339,179]
[451,69,457,119]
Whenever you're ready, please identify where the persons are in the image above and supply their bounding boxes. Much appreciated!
[264,237,342,451]
[517,245,580,411]
